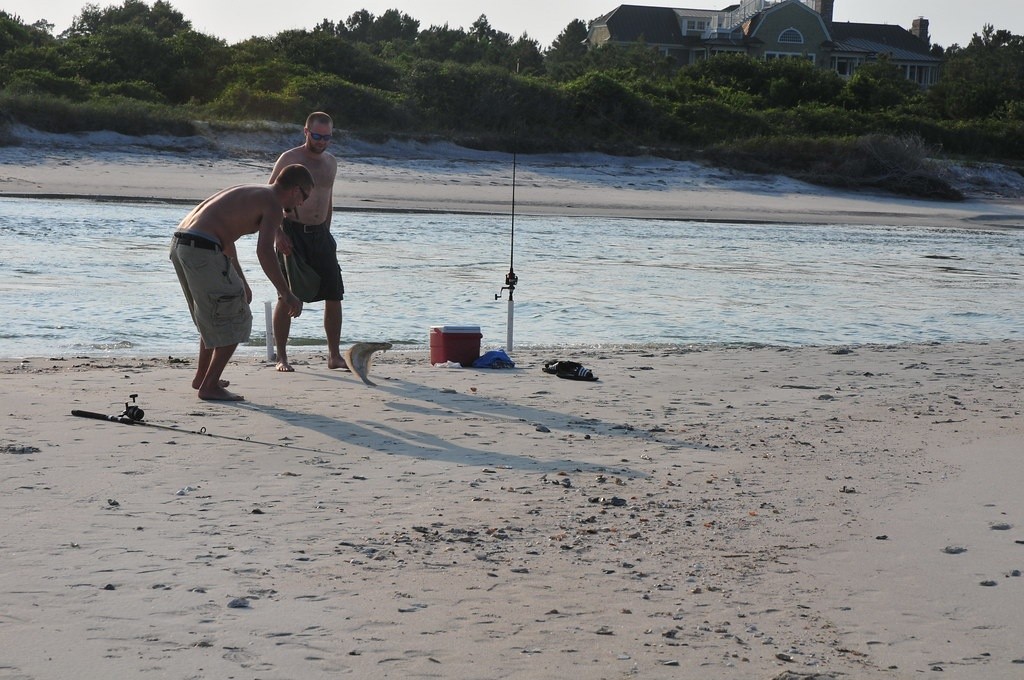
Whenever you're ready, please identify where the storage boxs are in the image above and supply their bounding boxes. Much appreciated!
[429,329,484,368]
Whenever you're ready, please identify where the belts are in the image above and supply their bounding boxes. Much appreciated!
[292,223,323,233]
[171,236,216,250]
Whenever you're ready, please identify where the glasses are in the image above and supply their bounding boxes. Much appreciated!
[295,184,309,201]
[308,130,332,140]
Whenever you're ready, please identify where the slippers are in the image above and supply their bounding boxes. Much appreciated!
[547,362,571,374]
[556,367,599,381]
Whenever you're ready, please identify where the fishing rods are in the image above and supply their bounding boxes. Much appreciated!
[70,392,345,456]
[495,59,521,354]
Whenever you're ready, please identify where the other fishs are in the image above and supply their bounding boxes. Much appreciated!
[344,341,393,387]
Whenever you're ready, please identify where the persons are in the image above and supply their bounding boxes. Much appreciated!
[169,164,315,402]
[269,112,350,372]
